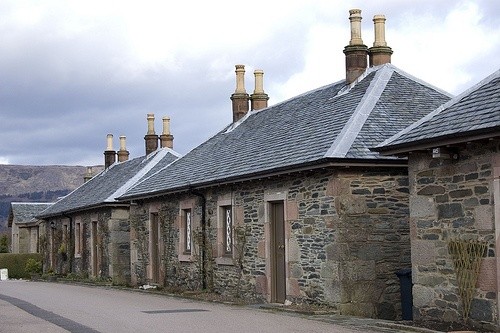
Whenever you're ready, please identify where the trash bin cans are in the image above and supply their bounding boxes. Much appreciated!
[396,269,412,321]
